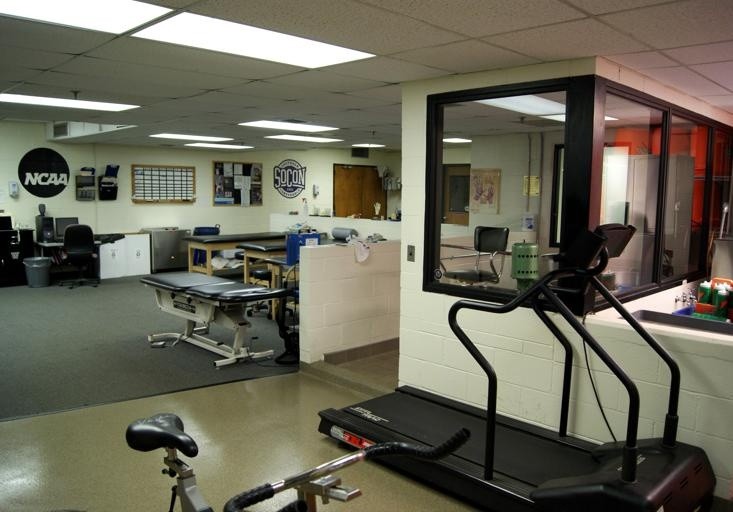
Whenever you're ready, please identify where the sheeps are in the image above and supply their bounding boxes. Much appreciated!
[681,295,691,305]
[689,291,697,303]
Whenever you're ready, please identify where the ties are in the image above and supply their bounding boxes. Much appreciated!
[56,218,78,237]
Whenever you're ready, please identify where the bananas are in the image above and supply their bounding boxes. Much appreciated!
[54,239,64,243]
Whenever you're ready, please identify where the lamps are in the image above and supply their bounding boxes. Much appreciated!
[58,227,100,289]
[440,226,511,287]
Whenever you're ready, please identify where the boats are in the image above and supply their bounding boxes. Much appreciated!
[39,204,45,216]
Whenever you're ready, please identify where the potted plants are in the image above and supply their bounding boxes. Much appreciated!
[211,159,262,207]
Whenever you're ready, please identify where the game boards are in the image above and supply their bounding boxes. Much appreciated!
[318,223,716,508]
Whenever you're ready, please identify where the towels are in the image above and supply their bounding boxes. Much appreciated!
[23,257,55,288]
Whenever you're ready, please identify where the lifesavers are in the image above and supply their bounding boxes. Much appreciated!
[36,216,54,243]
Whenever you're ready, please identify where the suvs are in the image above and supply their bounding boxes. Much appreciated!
[22,257,54,287]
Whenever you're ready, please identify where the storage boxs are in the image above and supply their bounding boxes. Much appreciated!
[139,272,290,368]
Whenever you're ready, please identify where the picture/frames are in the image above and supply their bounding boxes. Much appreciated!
[33,240,100,285]
[182,232,299,320]
